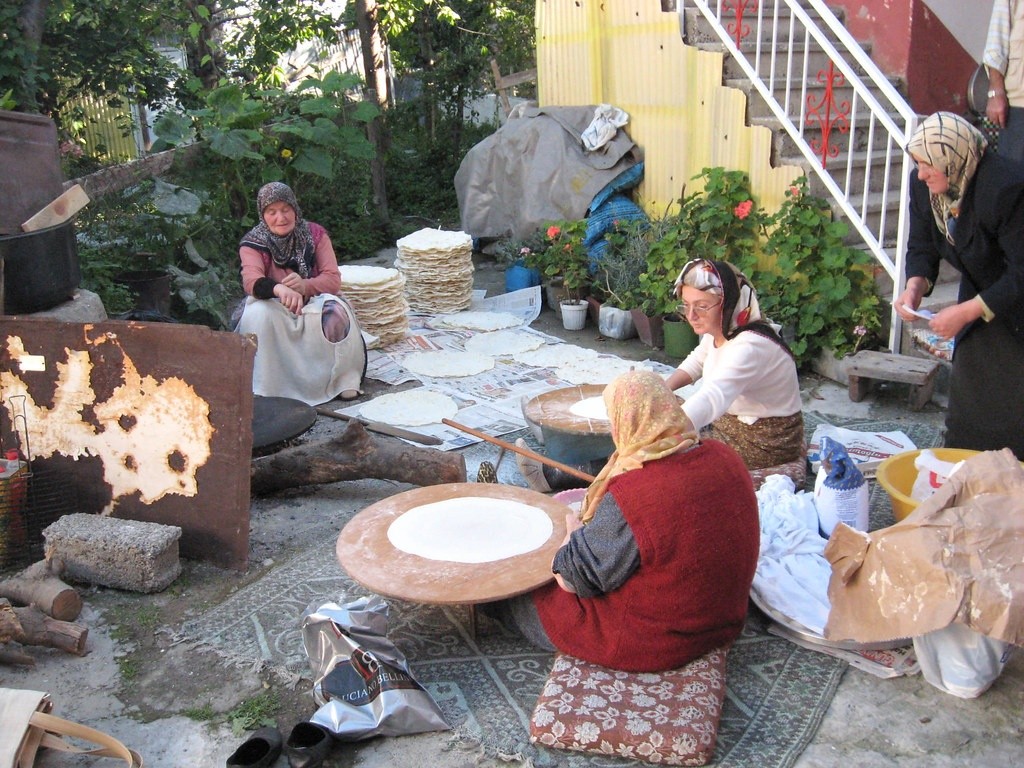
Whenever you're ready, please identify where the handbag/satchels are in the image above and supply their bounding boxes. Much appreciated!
[300,594,453,742]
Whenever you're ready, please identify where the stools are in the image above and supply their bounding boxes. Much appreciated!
[846,350,939,412]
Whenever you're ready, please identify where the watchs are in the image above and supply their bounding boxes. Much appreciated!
[987,88,1005,99]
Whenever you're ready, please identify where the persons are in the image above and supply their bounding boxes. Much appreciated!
[230,179,368,405]
[475,370,761,675]
[894,111,1024,466]
[518,256,808,492]
[984,0,1024,163]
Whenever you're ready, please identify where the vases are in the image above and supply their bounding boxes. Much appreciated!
[504,259,541,293]
[810,341,854,387]
[544,277,570,310]
[559,298,589,331]
[591,305,631,343]
[662,315,699,360]
[585,295,603,326]
[631,307,664,348]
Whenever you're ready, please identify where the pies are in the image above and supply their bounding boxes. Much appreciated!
[403,311,653,385]
[395,227,474,314]
[334,264,410,348]
[569,395,609,421]
[387,496,553,563]
[358,391,458,427]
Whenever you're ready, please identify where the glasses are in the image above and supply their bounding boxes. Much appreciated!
[676,301,721,315]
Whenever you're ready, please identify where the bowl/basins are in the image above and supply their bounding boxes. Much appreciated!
[876,448,1024,525]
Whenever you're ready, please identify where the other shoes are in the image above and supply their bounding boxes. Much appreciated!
[226,728,283,768]
[288,720,334,768]
[477,461,498,483]
[516,438,552,492]
[340,390,359,401]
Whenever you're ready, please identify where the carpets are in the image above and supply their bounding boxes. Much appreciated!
[174,529,849,768]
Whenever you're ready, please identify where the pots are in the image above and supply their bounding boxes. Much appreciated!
[0,216,81,315]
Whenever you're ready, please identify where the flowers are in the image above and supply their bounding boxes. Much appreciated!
[494,166,883,369]
